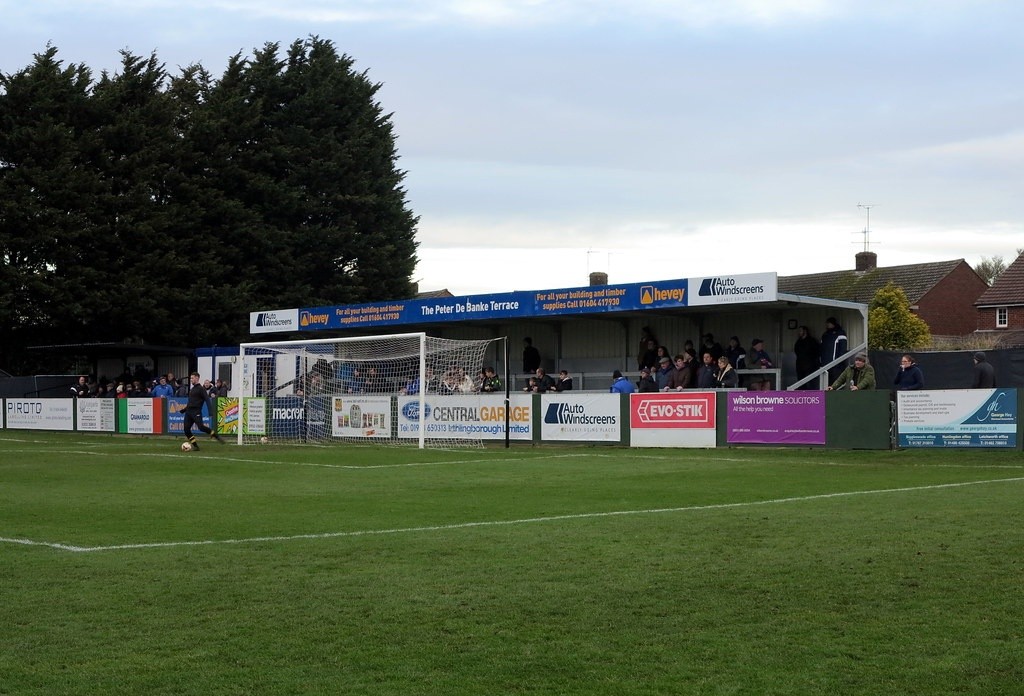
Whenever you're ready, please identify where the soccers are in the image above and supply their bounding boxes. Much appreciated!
[181,442,191,452]
[260,436,268,443]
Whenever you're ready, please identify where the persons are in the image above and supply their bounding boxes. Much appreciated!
[972,352,996,389]
[296,350,501,395]
[794,317,847,390]
[893,355,925,390]
[180,372,226,451]
[522,336,572,392]
[611,326,775,392]
[828,353,876,391]
[203,381,228,398]
[70,367,185,399]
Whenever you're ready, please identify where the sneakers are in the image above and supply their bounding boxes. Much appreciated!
[216,435,226,444]
[192,446,200,451]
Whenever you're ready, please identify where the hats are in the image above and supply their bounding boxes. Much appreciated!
[855,352,868,361]
[659,357,670,364]
[827,317,839,327]
[974,351,986,361]
[116,384,123,391]
[752,339,764,345]
[702,333,714,339]
